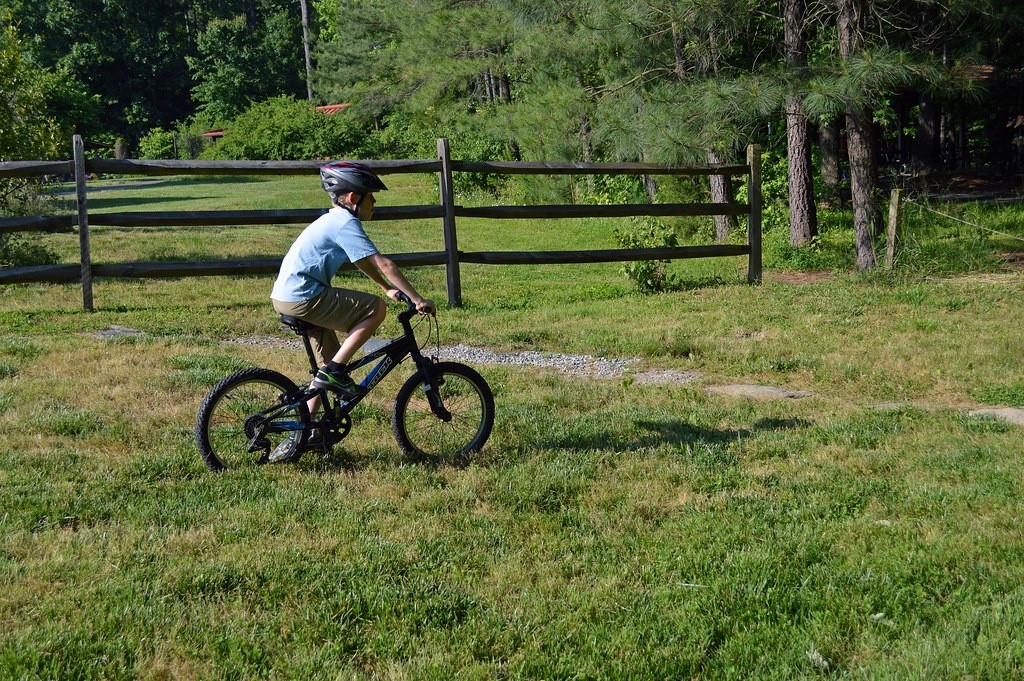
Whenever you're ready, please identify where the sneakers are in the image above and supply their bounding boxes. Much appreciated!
[289,430,321,449]
[313,366,359,400]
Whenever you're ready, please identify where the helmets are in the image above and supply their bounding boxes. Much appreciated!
[319,160,388,194]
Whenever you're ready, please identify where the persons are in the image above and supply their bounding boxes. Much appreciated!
[269,161,435,446]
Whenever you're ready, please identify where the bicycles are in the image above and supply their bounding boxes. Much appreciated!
[196,290,496,474]
